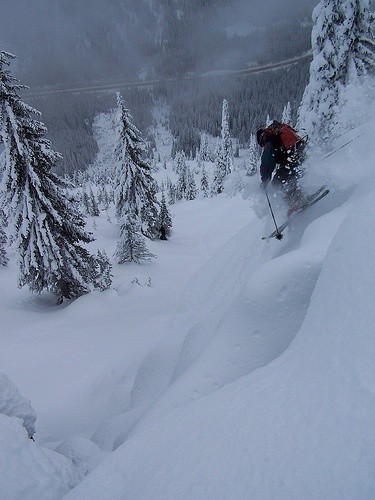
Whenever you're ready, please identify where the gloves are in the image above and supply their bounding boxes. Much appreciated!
[260,182,270,190]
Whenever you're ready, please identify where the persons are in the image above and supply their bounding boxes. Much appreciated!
[257,124,307,216]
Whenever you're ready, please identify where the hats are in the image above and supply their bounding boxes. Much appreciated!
[256,130,265,148]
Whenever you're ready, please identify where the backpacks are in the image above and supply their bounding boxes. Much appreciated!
[271,124,303,161]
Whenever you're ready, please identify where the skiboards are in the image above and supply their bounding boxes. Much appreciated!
[262,183,329,238]
